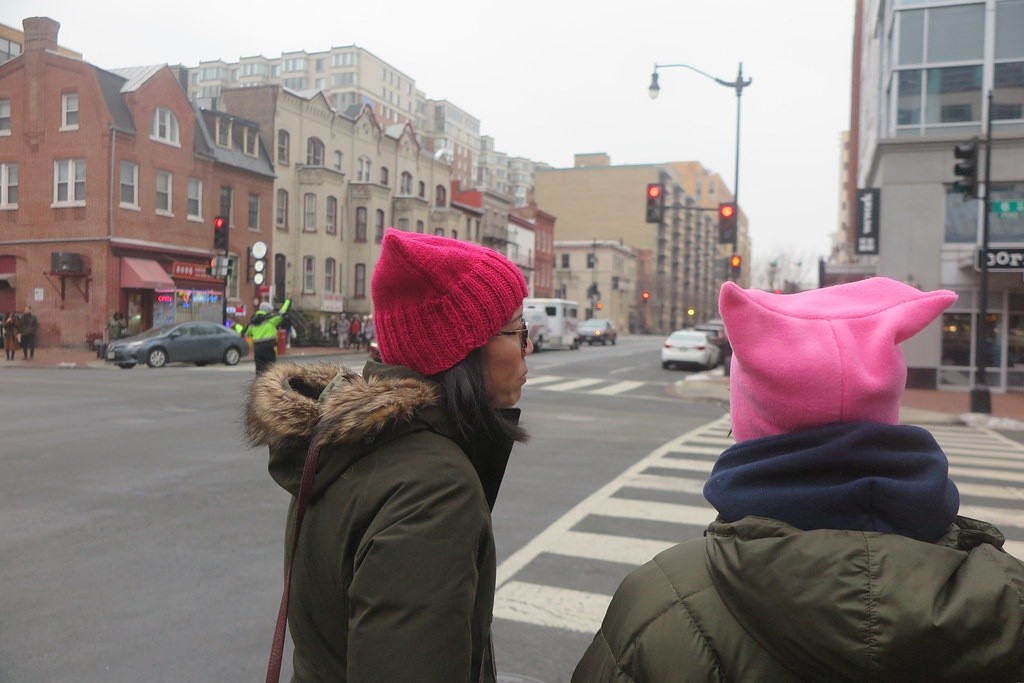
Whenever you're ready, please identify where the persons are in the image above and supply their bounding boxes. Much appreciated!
[242,286,293,379]
[331,312,376,349]
[17,305,37,360]
[232,319,244,334]
[240,227,534,683]
[571,278,1024,683]
[104,311,127,344]
[3,310,20,361]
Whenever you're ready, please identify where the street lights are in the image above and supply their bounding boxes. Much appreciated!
[649,65,742,285]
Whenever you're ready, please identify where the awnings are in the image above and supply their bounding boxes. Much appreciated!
[119,255,175,289]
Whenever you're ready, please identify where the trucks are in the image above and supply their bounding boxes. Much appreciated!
[524,298,579,351]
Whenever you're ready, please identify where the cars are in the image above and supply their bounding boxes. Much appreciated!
[660,330,722,371]
[697,318,728,357]
[103,321,249,369]
[579,320,616,344]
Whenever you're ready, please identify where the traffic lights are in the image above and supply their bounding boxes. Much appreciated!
[206,256,219,278]
[716,203,739,245]
[728,254,740,278]
[219,256,234,278]
[642,293,648,304]
[647,184,667,223]
[955,136,978,198]
[213,216,230,249]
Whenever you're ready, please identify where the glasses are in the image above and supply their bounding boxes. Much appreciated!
[493,317,528,357]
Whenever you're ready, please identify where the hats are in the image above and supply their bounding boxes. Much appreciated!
[259,302,274,314]
[718,277,959,446]
[370,227,529,379]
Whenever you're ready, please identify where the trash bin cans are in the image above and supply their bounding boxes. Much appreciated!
[97,343,108,359]
